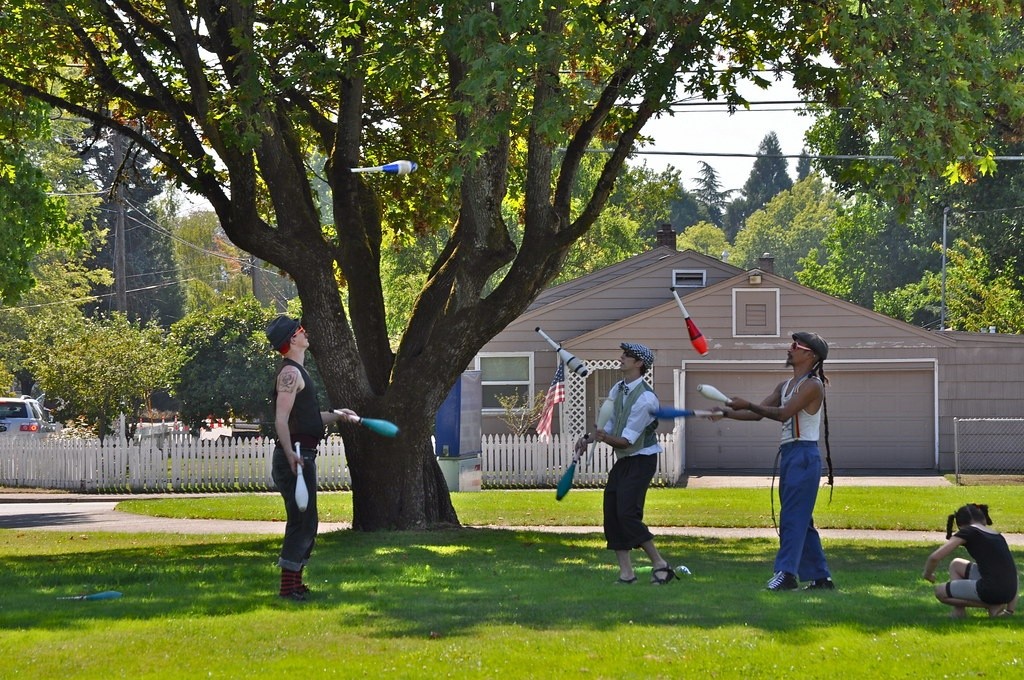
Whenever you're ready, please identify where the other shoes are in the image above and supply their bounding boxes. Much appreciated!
[279,584,313,601]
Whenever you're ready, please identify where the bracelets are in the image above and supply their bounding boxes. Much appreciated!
[583,433,591,439]
[1003,609,1013,615]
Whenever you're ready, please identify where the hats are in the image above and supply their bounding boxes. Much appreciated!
[266,314,300,352]
[620,342,654,369]
[792,332,829,360]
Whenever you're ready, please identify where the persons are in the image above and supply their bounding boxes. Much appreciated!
[922,503,1019,617]
[574,342,680,585]
[705,331,835,590]
[266,315,357,601]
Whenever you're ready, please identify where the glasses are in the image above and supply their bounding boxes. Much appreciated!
[623,350,640,360]
[287,325,304,343]
[792,341,816,358]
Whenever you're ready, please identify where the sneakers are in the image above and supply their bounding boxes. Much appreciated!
[762,570,799,592]
[802,577,835,591]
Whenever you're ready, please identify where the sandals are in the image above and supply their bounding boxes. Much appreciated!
[649,561,676,586]
[614,577,638,586]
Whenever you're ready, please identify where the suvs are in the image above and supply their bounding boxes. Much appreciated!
[0,394,51,453]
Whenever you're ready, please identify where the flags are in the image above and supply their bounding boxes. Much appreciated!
[535,362,565,441]
